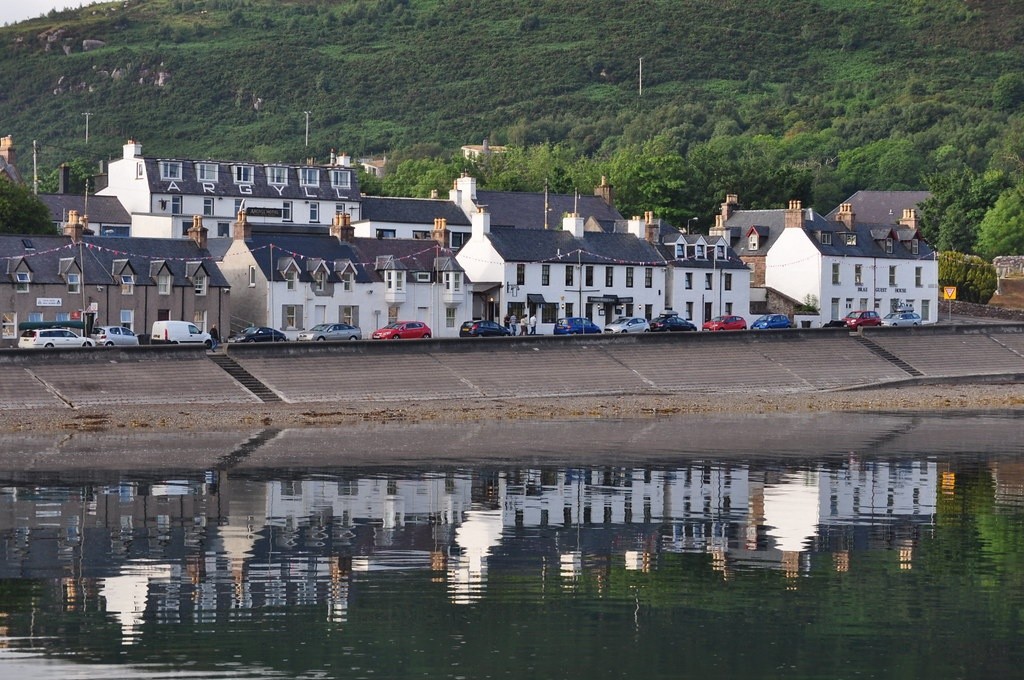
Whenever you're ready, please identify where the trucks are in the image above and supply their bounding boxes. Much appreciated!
[151,321,213,350]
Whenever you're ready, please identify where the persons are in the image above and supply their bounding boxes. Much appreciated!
[519,314,529,336]
[529,314,536,335]
[509,312,516,336]
[210,324,218,353]
[503,314,510,336]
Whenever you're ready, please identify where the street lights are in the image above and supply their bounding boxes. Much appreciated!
[688,217,698,236]
[304,111,312,146]
[81,113,94,143]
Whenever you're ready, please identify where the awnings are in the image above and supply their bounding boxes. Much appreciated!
[471,282,501,293]
[604,295,618,305]
[527,294,546,304]
[18,321,85,330]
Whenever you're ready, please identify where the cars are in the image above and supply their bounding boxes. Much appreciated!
[553,317,602,335]
[459,320,510,337]
[842,311,881,331]
[703,315,747,331]
[604,317,651,333]
[91,326,139,346]
[372,321,431,340]
[750,314,790,329]
[227,326,286,343]
[298,323,362,341]
[649,317,697,332]
[880,312,922,327]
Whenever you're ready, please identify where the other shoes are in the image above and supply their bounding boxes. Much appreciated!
[211,348,215,352]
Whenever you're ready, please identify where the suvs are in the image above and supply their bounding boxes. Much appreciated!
[18,329,95,348]
